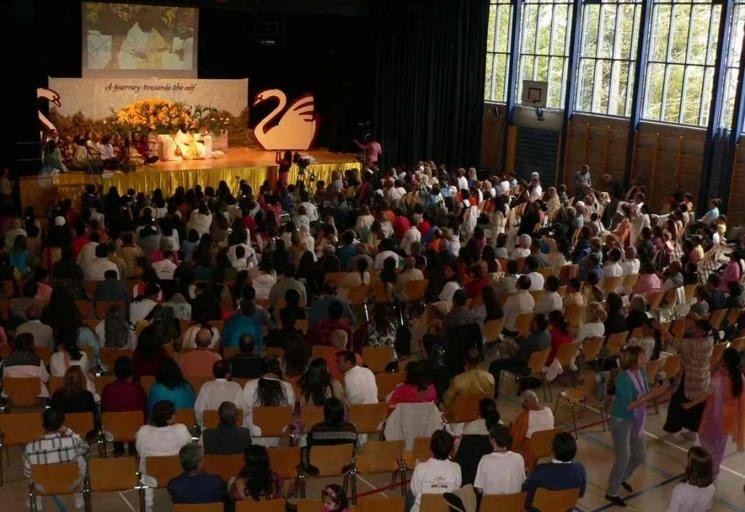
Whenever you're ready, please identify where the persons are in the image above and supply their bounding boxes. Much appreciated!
[1,126,744,511]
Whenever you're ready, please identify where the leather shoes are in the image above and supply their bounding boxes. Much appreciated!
[621,482,633,494]
[605,494,627,507]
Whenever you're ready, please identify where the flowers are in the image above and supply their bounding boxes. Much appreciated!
[107,96,206,136]
